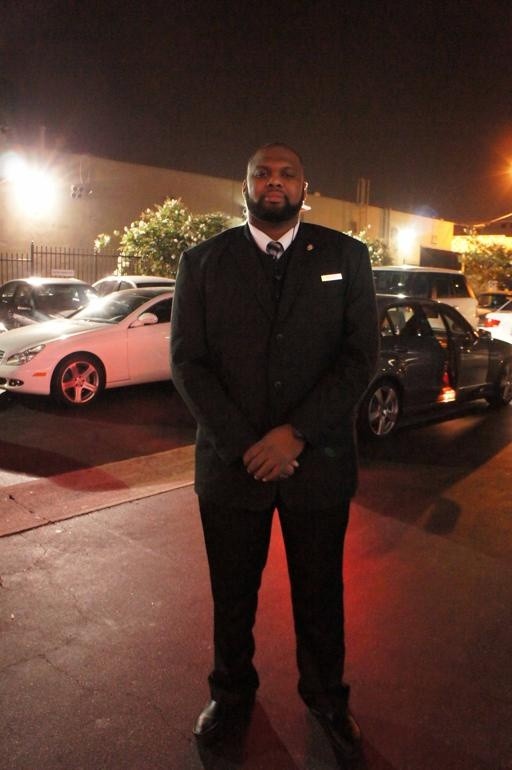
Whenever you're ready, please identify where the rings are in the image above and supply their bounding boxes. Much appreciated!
[279,474,288,481]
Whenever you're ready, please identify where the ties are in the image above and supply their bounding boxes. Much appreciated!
[265,242,285,270]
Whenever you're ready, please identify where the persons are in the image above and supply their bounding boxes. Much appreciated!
[168,142,378,761]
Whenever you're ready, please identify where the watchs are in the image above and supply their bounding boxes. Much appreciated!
[288,424,305,441]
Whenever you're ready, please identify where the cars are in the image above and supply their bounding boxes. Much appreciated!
[1,273,178,403]
[357,265,511,437]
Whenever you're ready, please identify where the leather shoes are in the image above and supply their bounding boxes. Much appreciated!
[301,699,365,756]
[191,684,258,738]
